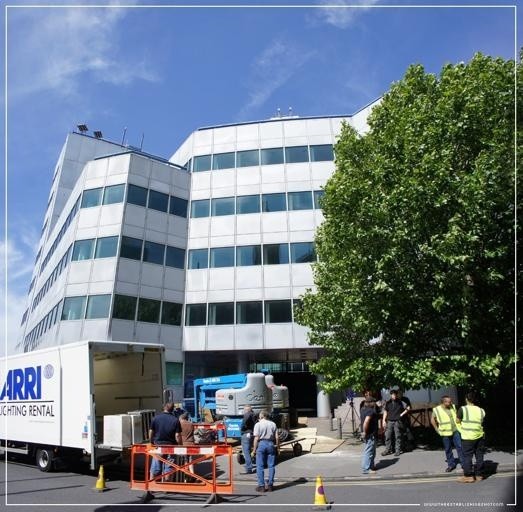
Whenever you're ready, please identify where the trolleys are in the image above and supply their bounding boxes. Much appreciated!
[236,436,307,466]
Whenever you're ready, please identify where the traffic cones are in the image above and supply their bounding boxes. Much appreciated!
[91,465,112,492]
[308,474,337,506]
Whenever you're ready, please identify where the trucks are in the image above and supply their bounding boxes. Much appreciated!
[0,338,167,473]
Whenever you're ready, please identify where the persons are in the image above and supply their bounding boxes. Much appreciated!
[147,401,194,496]
[430,396,465,472]
[358,389,412,475]
[240,405,256,474]
[456,392,487,483]
[251,411,281,492]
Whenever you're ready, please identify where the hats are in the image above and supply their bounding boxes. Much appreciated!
[365,397,377,402]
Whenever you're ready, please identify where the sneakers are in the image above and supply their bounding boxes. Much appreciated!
[256,485,273,492]
[362,466,381,474]
[445,465,484,483]
[239,468,252,475]
[381,449,405,457]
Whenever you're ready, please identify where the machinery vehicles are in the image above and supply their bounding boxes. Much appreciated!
[188,371,291,447]
